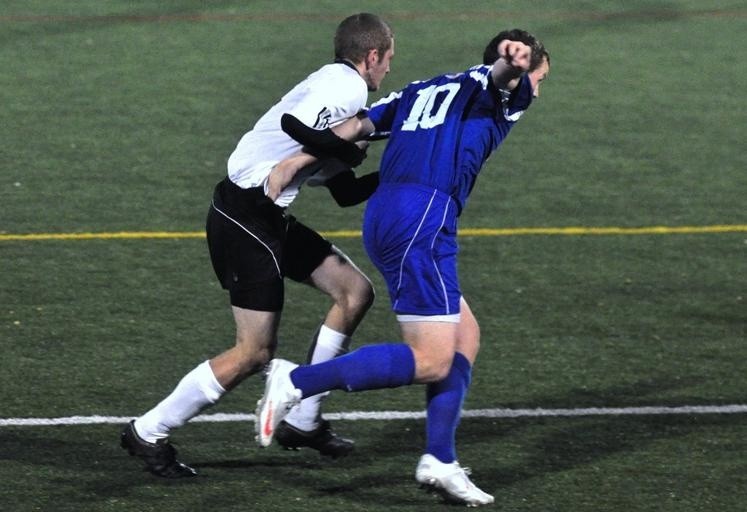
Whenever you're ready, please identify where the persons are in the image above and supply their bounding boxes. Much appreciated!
[255,29,550,508]
[120,15,395,479]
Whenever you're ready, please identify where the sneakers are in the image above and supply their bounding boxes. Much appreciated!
[416,455,495,506]
[274,418,354,456]
[255,358,303,448]
[120,419,195,478]
